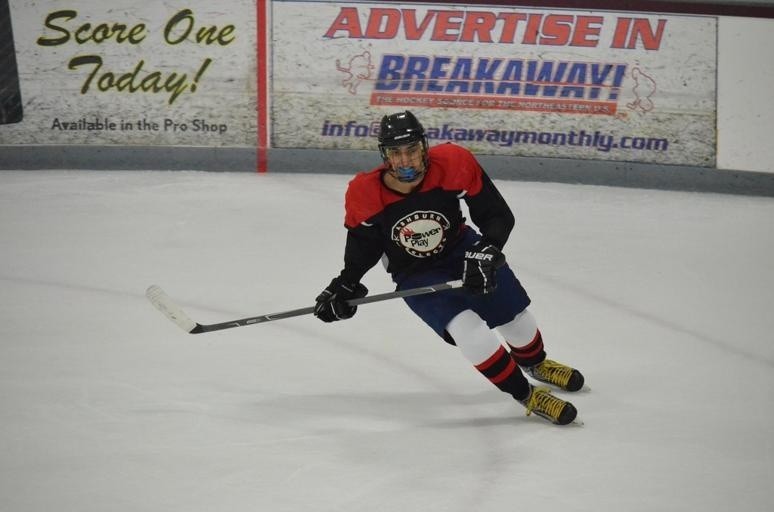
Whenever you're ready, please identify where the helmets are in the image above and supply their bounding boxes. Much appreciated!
[377,110,429,185]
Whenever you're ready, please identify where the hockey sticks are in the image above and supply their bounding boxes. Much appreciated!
[145,279,466,334]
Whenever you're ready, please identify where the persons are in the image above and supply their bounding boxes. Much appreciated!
[311,107,586,425]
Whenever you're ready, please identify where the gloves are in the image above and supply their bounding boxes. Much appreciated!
[450,239,506,297]
[313,276,369,323]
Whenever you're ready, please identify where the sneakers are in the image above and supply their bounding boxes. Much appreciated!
[517,382,577,426]
[527,359,584,392]
